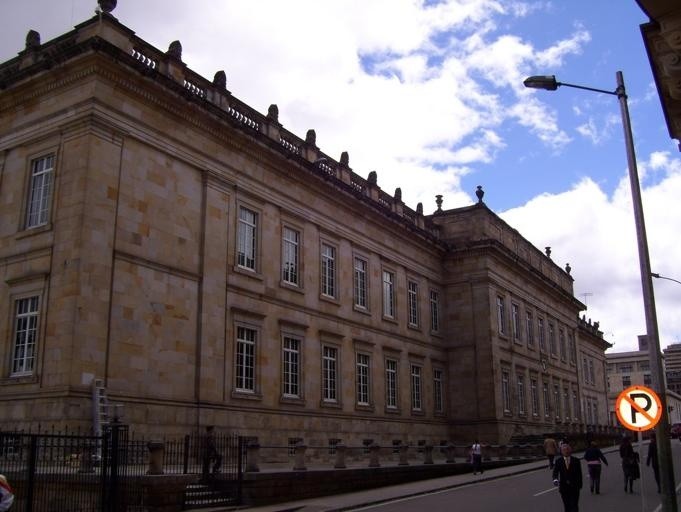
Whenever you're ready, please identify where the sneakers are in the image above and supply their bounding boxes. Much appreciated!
[590,489,600,494]
[624,488,632,492]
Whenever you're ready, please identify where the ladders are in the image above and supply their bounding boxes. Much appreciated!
[92,378,110,464]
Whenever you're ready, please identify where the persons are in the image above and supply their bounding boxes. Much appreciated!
[472,439,484,475]
[199,425,222,482]
[552,444,583,512]
[584,440,608,494]
[620,435,637,494]
[646,431,661,493]
[670,424,681,442]
[543,434,557,469]
[0,473,16,512]
[559,438,563,455]
[586,427,594,447]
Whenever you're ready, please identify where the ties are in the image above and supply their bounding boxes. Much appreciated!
[566,456,569,469]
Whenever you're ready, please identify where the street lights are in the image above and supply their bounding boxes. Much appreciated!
[523,71,677,512]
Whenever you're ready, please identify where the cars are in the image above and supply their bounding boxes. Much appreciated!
[670,423,681,439]
